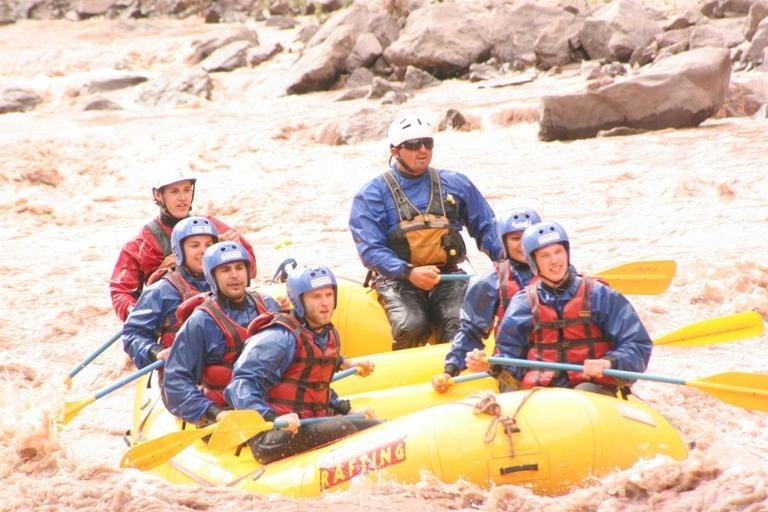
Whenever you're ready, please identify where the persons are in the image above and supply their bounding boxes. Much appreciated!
[109,162,257,371]
[162,240,375,447]
[119,216,293,392]
[348,108,508,353]
[222,263,381,468]
[434,207,611,395]
[465,219,652,398]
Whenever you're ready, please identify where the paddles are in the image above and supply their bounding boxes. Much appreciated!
[445,310,765,388]
[437,260,677,296]
[57,360,164,425]
[205,409,371,453]
[120,366,362,472]
[475,348,768,413]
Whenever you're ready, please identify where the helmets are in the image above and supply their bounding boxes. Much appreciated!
[498,205,568,274]
[147,158,199,191]
[285,262,339,320]
[200,240,253,296]
[170,213,219,267]
[389,109,436,148]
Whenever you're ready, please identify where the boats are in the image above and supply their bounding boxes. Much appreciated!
[129,268,691,501]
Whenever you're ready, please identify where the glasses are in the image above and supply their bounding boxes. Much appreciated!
[399,140,434,150]
